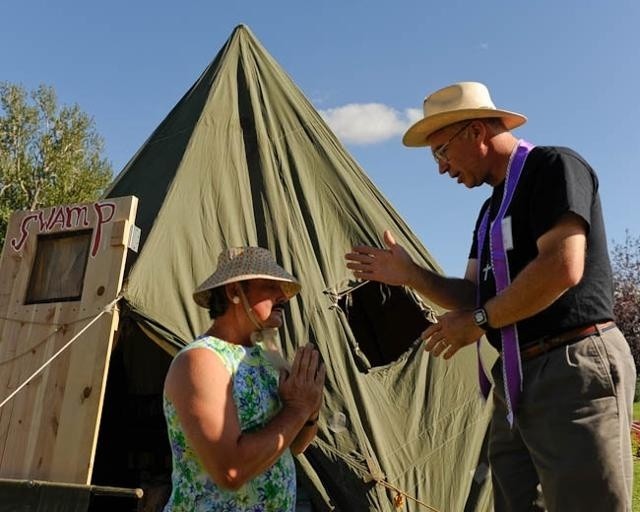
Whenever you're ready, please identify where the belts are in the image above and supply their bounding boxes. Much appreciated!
[520,318,616,359]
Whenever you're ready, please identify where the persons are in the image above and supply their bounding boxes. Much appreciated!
[346,78,635,509]
[163,246,328,510]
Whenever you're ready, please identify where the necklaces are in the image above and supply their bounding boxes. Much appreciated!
[481,263,492,281]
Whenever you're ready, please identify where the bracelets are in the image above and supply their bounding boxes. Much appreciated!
[303,415,319,426]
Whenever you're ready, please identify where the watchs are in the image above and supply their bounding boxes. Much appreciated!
[473,301,497,334]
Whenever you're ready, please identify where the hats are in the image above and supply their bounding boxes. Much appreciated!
[192,246,302,309]
[402,82,527,148]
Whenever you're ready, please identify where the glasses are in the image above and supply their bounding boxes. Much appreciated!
[433,121,480,164]
